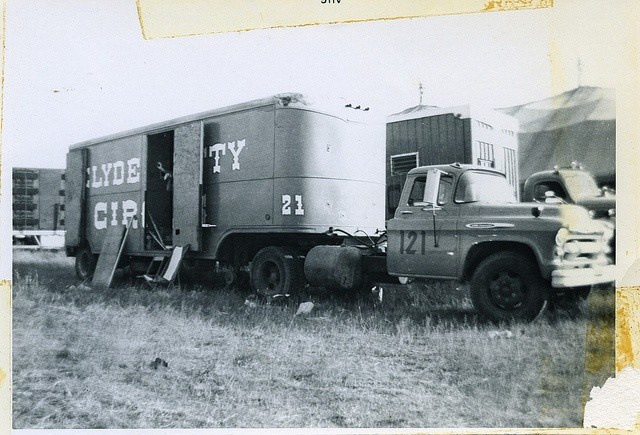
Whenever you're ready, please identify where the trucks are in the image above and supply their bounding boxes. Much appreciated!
[65,92,615,325]
[386,105,615,224]
